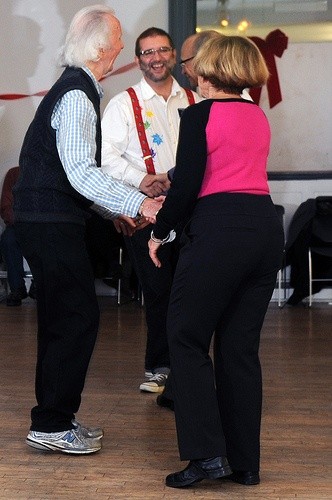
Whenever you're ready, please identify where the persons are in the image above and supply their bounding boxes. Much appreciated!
[0,167,36,306]
[146,29,252,411]
[102,26,207,392]
[144,36,285,488]
[13,7,162,453]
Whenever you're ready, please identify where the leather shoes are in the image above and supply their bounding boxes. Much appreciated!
[166,452,232,487]
[229,469,259,486]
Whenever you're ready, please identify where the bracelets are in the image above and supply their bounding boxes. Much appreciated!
[151,231,170,243]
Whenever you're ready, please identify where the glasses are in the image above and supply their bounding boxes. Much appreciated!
[139,47,174,57]
[180,57,195,70]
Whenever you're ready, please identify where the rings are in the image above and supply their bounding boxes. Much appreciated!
[120,222,124,224]
[156,211,158,215]
[136,216,141,224]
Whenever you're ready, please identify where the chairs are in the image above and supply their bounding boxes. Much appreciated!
[276,204,287,309]
[301,195,332,307]
[88,210,125,306]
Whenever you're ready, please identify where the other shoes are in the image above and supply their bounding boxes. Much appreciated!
[28,281,38,298]
[7,286,27,306]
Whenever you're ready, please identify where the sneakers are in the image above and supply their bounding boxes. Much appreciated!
[25,412,103,454]
[139,367,166,393]
